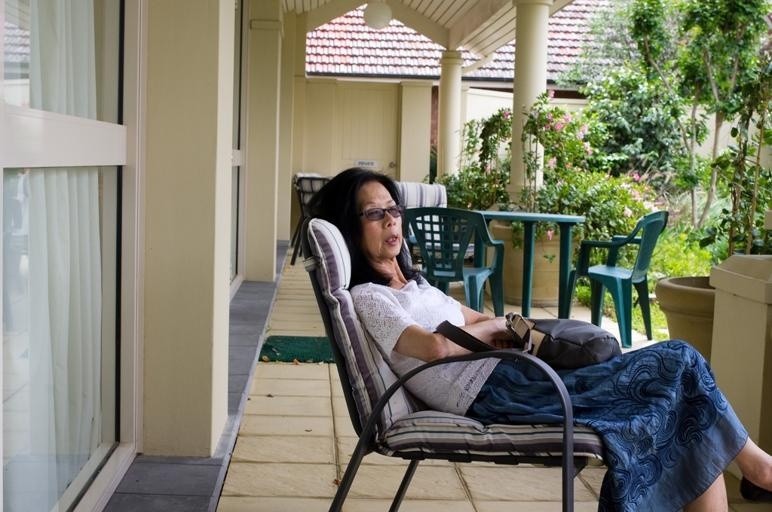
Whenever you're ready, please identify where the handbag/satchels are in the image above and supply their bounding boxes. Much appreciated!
[505,312,621,368]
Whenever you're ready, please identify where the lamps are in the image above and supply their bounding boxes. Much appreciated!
[364,0,393,30]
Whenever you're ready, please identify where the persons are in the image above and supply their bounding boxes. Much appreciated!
[304,166,772,511]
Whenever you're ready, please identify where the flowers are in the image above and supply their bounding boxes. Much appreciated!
[480,90,655,219]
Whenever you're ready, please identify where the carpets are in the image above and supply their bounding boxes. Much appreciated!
[257,334,336,365]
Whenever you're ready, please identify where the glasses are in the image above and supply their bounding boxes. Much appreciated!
[359,204,405,221]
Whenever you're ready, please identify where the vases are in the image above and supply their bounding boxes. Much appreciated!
[655,275,714,366]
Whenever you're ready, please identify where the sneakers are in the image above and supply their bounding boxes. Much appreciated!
[739,475,772,504]
